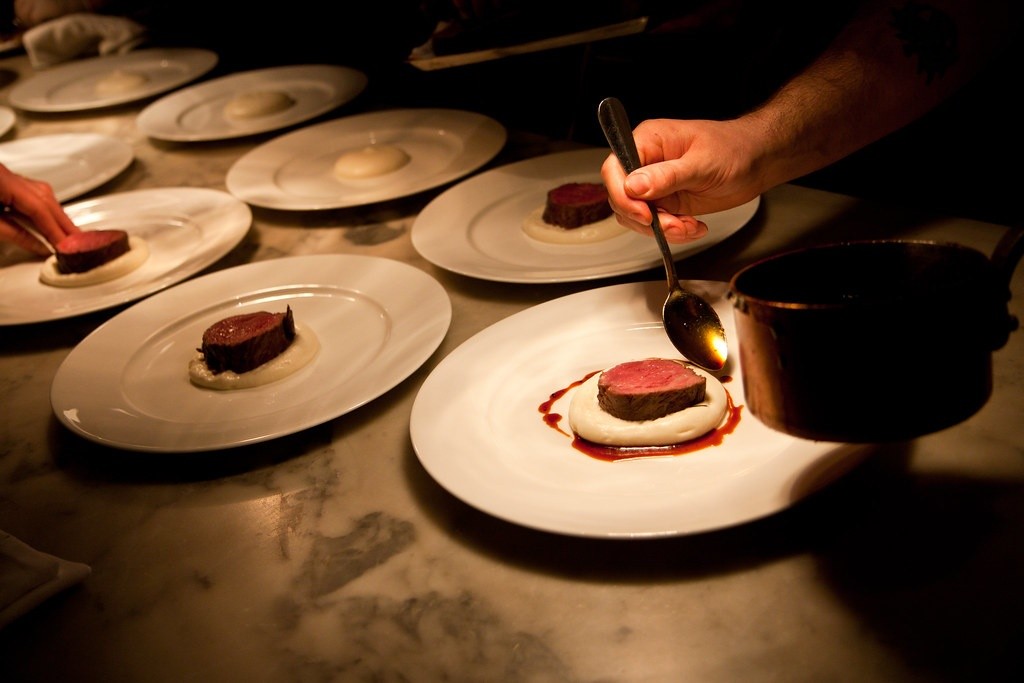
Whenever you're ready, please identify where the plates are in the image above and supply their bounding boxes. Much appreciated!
[409,280,869,537]
[411,148,760,282]
[225,110,506,210]
[0,132,133,203]
[0,187,253,325]
[0,50,217,137]
[137,65,369,140]
[50,253,452,452]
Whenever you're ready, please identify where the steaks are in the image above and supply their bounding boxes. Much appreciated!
[199,303,295,374]
[596,358,707,422]
[54,229,129,273]
[541,181,612,229]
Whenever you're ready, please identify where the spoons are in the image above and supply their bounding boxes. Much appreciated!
[598,96,728,371]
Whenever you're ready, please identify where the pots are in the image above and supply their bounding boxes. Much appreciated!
[727,216,1024,444]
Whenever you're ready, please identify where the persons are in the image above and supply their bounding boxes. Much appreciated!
[601,0,1024,244]
